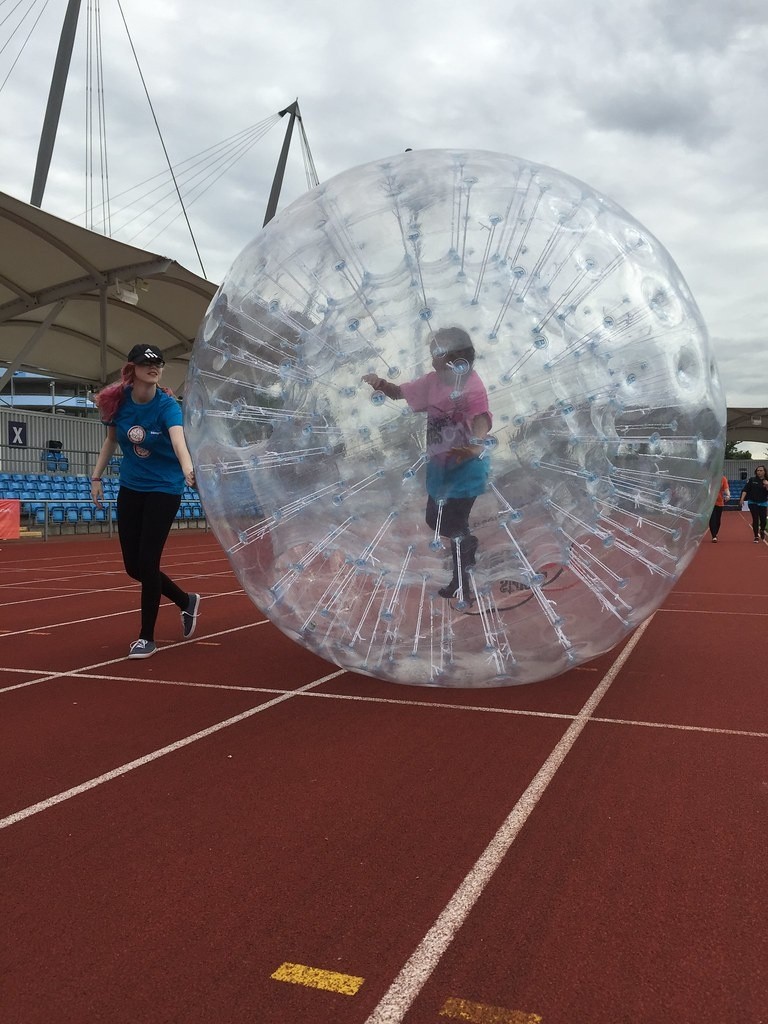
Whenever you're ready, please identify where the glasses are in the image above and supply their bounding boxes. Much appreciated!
[134,359,165,368]
[757,470,765,472]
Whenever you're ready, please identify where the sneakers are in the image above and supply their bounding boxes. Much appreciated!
[760,530,765,540]
[712,537,717,543]
[754,538,759,543]
[181,592,201,637]
[127,638,157,659]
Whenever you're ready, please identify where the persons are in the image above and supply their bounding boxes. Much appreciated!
[740,465,768,544]
[361,326,493,599]
[708,475,731,544]
[90,343,200,660]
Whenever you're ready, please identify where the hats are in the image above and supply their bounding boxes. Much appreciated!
[128,345,164,364]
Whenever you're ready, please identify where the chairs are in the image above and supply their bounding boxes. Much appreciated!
[723,480,749,509]
[0,452,269,537]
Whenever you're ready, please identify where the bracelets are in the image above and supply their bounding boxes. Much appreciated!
[91,478,101,481]
